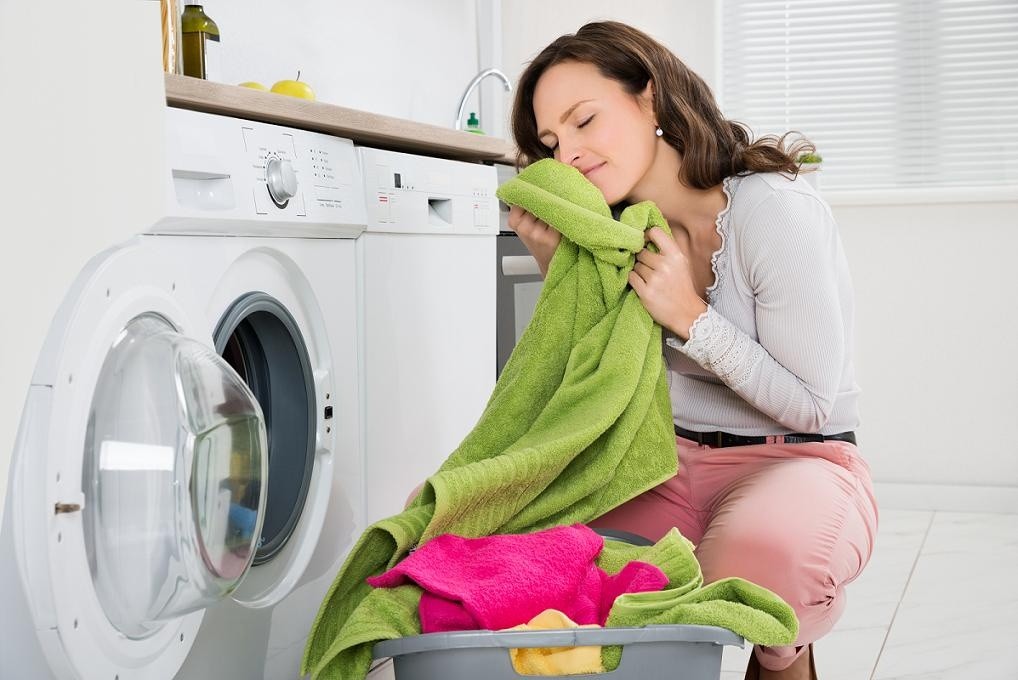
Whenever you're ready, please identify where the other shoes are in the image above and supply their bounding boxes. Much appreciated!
[745,639,819,680]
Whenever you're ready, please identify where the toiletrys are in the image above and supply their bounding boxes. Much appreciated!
[464,112,483,135]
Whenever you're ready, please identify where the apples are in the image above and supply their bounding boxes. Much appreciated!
[241,70,315,99]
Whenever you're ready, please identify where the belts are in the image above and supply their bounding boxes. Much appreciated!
[674,424,857,449]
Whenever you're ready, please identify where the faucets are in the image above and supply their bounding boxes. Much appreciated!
[453,67,513,134]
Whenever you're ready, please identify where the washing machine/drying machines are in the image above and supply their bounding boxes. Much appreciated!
[351,136,504,531]
[13,103,378,680]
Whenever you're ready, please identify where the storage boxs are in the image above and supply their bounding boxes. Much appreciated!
[374,624,746,679]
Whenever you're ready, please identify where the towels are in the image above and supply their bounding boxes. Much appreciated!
[300,158,683,679]
[500,611,606,678]
[590,528,798,672]
[366,525,667,631]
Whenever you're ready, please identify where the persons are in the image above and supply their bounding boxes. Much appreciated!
[503,20,882,678]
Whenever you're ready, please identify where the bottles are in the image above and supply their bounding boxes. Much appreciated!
[179,0,224,82]
